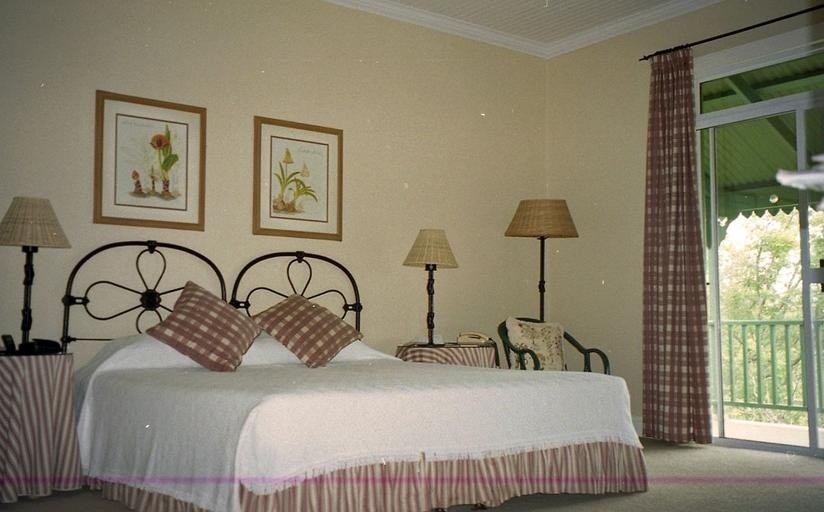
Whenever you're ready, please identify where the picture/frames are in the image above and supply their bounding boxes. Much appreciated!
[251,115,344,240]
[91,86,208,232]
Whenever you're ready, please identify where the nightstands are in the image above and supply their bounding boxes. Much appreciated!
[0,346,74,503]
[396,333,494,370]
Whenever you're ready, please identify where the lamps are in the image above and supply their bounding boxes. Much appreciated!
[1,195,71,355]
[402,225,458,348]
[505,198,581,321]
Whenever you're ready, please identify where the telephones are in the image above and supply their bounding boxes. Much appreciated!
[457,332,492,347]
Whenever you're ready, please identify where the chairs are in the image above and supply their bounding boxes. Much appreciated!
[491,315,610,375]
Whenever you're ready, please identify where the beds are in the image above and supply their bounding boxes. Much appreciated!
[60,238,633,510]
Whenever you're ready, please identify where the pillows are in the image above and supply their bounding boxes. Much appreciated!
[144,275,365,370]
[503,317,566,373]
